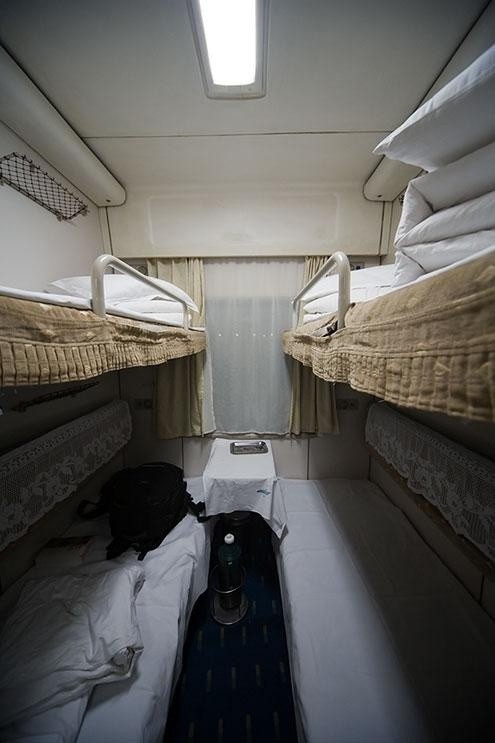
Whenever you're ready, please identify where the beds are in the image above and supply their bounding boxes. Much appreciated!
[270,250,495,743]
[0,253,214,742]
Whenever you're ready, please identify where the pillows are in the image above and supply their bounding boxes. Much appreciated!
[46,267,200,312]
[301,264,396,300]
[371,47,494,173]
[303,288,394,313]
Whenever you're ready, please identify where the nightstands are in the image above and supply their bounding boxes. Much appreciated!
[201,438,274,518]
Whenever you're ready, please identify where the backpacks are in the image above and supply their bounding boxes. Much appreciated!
[77,461,205,562]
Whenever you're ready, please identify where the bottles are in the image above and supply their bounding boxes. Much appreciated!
[217,531,243,611]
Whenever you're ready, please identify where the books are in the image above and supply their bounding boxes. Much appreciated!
[33,534,94,567]
